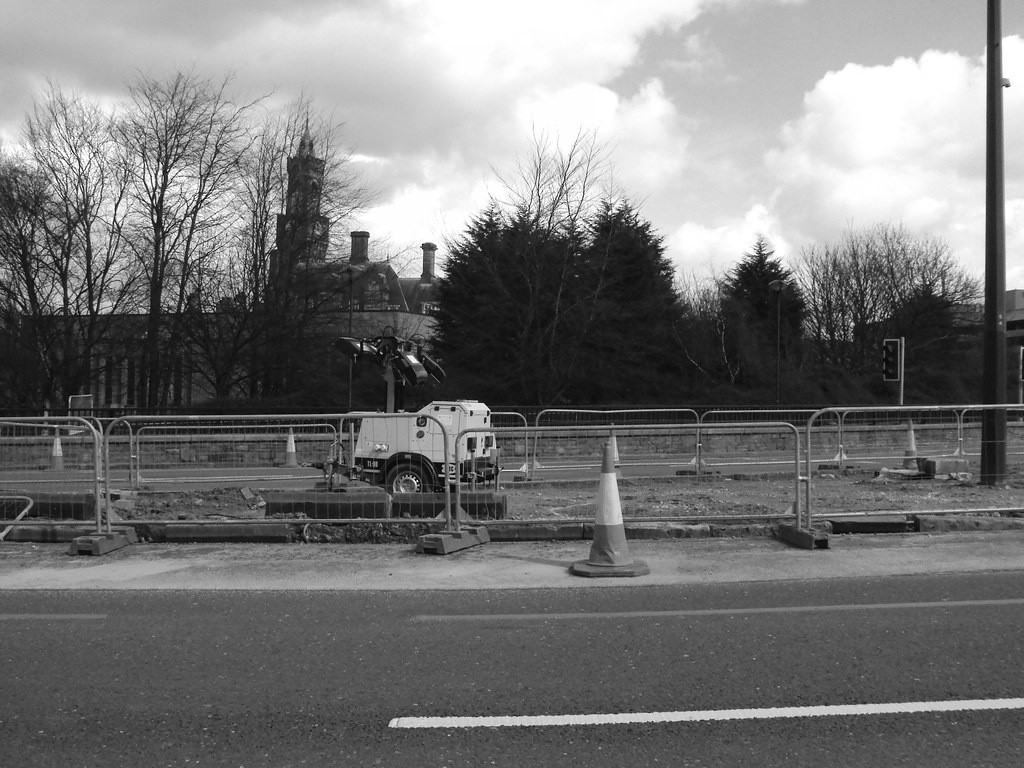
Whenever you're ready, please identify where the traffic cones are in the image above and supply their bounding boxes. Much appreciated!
[44,428,66,472]
[279,428,300,468]
[608,423,621,468]
[568,441,651,577]
[895,418,919,469]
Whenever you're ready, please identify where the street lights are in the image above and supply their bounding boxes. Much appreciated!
[768,280,787,404]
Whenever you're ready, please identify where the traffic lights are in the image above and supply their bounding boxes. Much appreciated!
[882,338,900,381]
